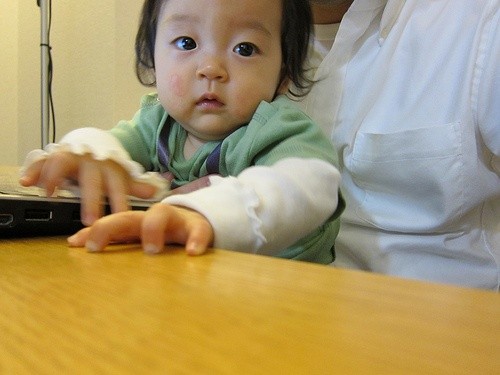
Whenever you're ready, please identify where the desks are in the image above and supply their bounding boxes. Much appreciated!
[1,236,499,375]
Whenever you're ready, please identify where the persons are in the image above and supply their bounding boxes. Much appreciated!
[20,1,346,265]
[282,0,500,288]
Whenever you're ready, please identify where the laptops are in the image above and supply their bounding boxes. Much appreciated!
[0,188,157,243]
